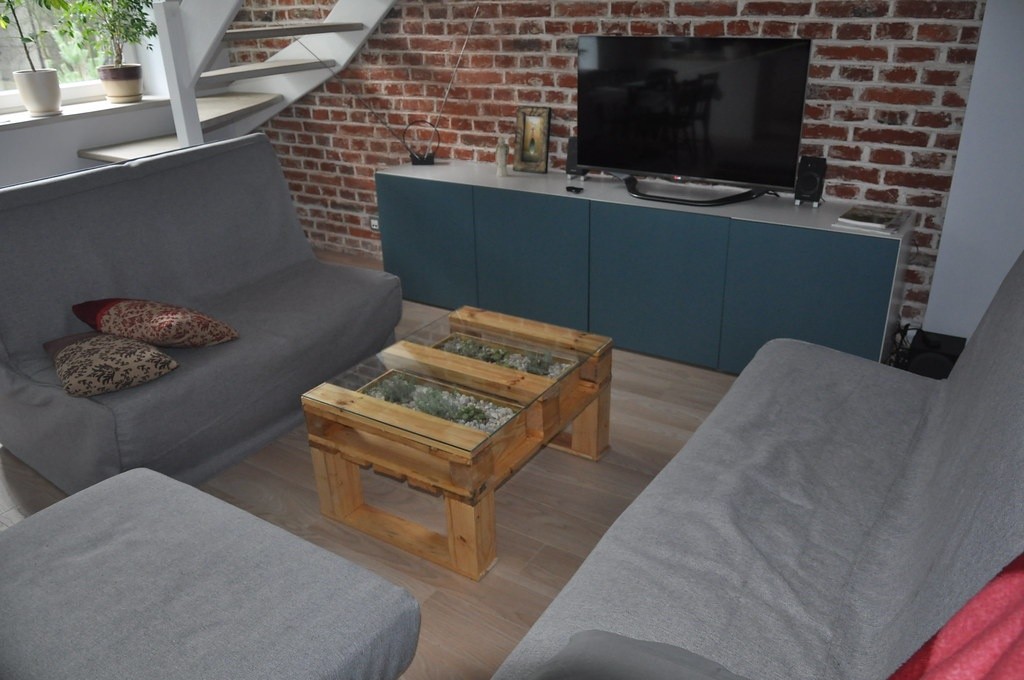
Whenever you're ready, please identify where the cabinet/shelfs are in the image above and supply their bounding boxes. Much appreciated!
[374,158,917,378]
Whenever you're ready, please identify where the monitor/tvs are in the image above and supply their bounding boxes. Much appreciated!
[577,34,811,207]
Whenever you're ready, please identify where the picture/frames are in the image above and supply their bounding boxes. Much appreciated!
[513,107,551,173]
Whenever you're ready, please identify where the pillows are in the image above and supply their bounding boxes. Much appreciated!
[68,298,239,349]
[44,332,177,397]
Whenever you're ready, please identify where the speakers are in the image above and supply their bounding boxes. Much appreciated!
[566,136,588,181]
[795,156,827,208]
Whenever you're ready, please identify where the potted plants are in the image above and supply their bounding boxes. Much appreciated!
[0,0,68,117]
[60,0,159,104]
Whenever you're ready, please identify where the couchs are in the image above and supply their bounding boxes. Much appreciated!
[0,133,403,495]
[0,468,422,680]
[492,251,1024,680]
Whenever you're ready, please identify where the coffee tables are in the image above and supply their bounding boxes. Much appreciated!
[301,306,611,583]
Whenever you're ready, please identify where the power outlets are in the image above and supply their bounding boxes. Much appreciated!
[369,216,380,231]
[896,319,917,348]
